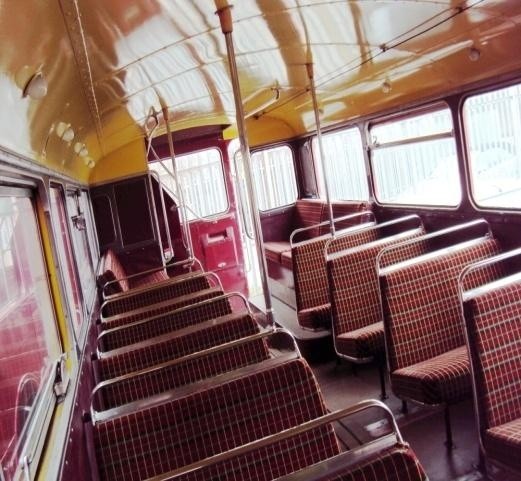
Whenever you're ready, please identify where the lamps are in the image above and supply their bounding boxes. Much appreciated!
[14,65,35,90]
[380,82,391,94]
[74,143,82,153]
[59,123,75,142]
[56,121,66,136]
[76,147,89,158]
[20,71,48,100]
[466,47,481,60]
[87,160,96,168]
[84,157,91,165]
